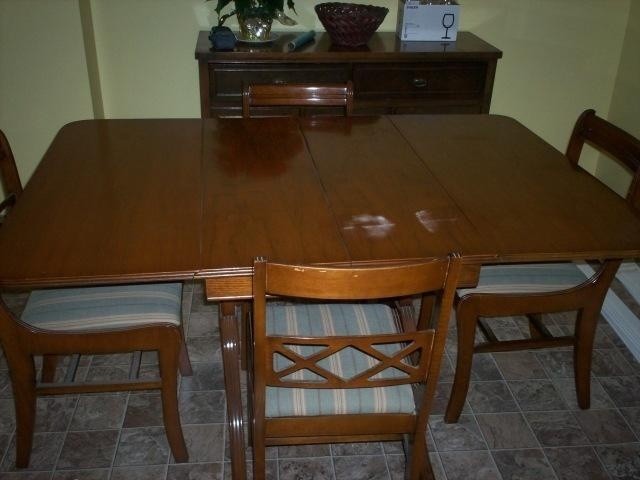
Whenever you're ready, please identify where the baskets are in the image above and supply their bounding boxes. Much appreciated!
[313,2,390,48]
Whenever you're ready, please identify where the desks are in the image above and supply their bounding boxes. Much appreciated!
[0,115,640,479]
[195,29,503,114]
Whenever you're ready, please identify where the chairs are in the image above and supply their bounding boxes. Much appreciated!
[0,127,189,467]
[443,109,640,424]
[242,78,355,117]
[245,253,459,479]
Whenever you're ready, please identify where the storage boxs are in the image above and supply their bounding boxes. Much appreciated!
[397,2,459,41]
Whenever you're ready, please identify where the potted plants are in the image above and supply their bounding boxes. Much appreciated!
[215,1,297,38]
